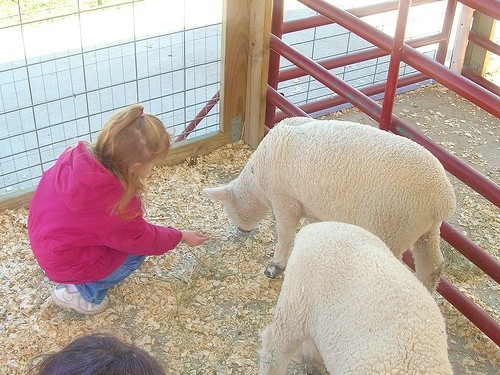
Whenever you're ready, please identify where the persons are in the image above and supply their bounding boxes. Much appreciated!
[28,106,210,315]
[26,333,168,375]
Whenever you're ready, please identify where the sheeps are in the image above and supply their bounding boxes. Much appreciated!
[203,115,457,296]
[257,222,456,375]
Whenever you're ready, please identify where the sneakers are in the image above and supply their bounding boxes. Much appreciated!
[52,282,108,315]
